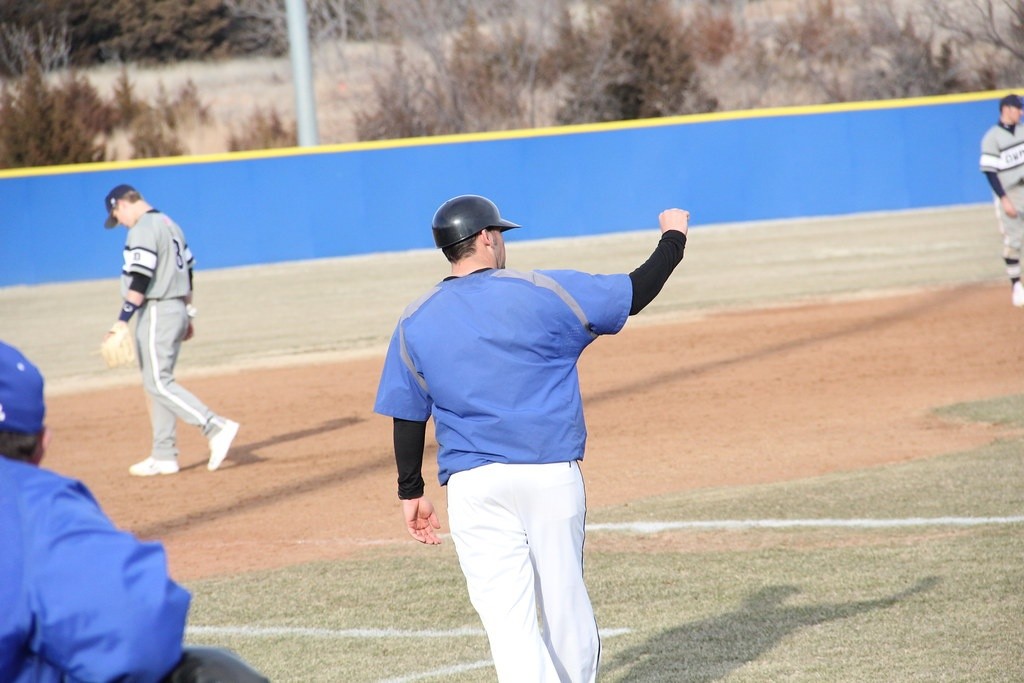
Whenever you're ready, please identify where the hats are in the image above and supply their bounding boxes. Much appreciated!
[103,184,135,229]
[1000,95,1024,109]
[0,342,45,432]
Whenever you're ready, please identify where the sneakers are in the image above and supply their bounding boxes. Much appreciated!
[129,456,180,477]
[208,420,240,471]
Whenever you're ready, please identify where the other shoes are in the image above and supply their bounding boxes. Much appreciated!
[1012,282,1024,307]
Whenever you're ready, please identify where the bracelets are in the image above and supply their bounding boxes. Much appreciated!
[186,304,195,316]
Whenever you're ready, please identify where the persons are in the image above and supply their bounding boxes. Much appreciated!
[373,195,688,683]
[980,94,1024,308]
[0,341,267,682]
[104,184,240,476]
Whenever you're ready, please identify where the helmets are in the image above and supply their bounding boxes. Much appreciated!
[432,194,523,248]
